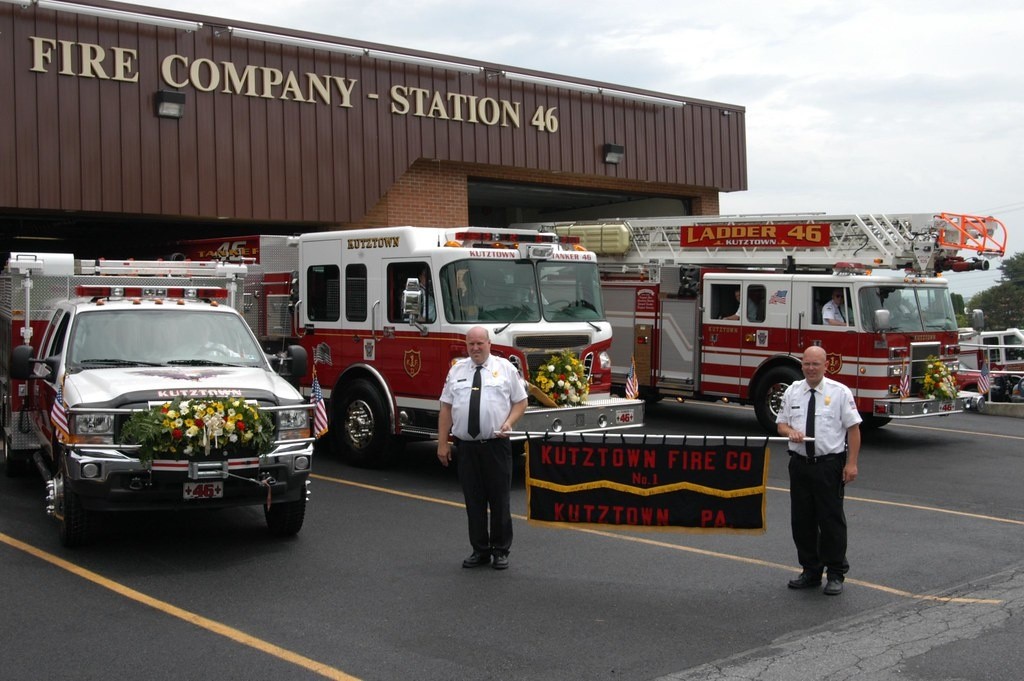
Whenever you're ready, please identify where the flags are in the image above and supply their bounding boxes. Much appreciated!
[977,360,990,394]
[900,371,909,400]
[51,385,70,445]
[625,354,639,399]
[310,372,330,438]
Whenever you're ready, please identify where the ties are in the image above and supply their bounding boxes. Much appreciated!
[468,366,484,439]
[838,306,846,323]
[806,389,816,458]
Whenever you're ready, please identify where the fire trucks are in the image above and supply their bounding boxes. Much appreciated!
[0,250,316,550]
[168,222,648,468]
[954,327,1024,402]
[510,209,1010,436]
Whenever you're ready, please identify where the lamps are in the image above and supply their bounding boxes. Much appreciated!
[0,0,203,33]
[154,89,188,118]
[603,144,626,165]
[488,70,687,110]
[216,26,484,77]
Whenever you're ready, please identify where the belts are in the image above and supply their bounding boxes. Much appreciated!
[797,454,822,464]
[465,438,500,444]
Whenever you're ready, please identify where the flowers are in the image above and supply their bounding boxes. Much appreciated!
[116,391,276,466]
[533,352,587,407]
[920,356,961,400]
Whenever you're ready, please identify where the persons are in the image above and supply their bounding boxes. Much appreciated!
[175,318,242,358]
[776,346,864,595]
[460,271,491,305]
[721,288,757,322]
[509,266,550,305]
[437,327,529,569]
[821,288,854,326]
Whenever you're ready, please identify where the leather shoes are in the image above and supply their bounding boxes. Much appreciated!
[493,553,508,569]
[788,572,822,588]
[824,578,844,594]
[463,552,491,568]
